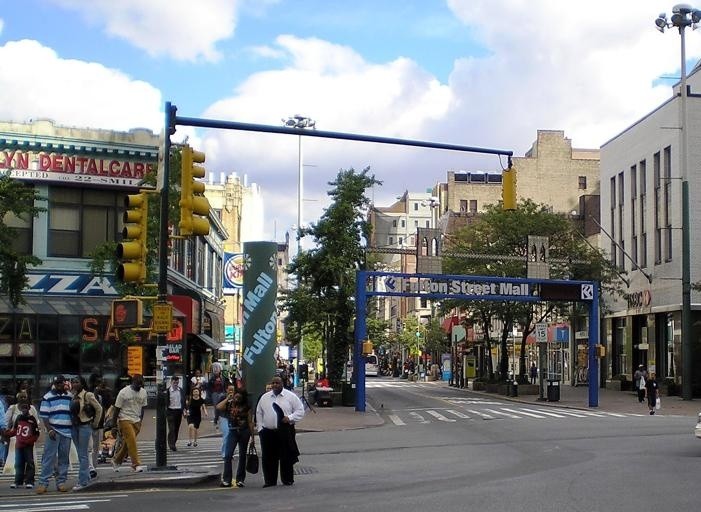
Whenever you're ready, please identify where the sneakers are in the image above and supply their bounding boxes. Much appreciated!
[135,466,143,472]
[97,457,106,464]
[170,446,176,451]
[194,442,198,446]
[650,412,653,415]
[10,472,97,494]
[187,442,191,446]
[643,399,645,401]
[639,401,642,403]
[111,460,120,472]
[263,485,276,488]
[282,481,294,486]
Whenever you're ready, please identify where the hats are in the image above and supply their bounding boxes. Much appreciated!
[53,374,65,384]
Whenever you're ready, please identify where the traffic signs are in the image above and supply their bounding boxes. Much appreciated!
[535,324,546,342]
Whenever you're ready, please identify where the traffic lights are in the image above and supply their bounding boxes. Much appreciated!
[415,326,420,337]
[114,191,148,287]
[596,347,602,357]
[110,298,143,328]
[179,144,212,237]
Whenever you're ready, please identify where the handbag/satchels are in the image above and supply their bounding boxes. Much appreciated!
[82,403,95,418]
[246,442,259,473]
[221,482,232,487]
[656,399,660,409]
[236,482,244,487]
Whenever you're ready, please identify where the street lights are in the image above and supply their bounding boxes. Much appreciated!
[281,113,315,376]
[654,4,699,400]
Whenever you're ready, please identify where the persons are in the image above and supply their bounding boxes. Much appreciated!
[276,357,296,392]
[255,375,306,489]
[645,373,660,415]
[529,363,538,384]
[633,364,648,403]
[255,383,273,427]
[0,357,256,497]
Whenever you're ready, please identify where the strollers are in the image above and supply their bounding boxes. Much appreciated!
[97,416,127,464]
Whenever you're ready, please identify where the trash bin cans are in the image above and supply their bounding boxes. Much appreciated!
[342,381,356,406]
[546,379,561,401]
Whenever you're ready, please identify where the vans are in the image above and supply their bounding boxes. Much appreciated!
[364,354,380,376]
